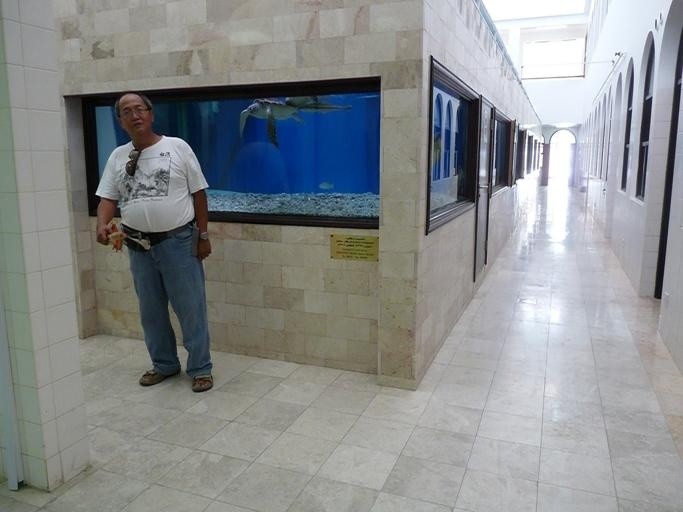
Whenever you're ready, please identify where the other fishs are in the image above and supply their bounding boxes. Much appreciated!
[318,182,334,192]
[297,103,352,113]
[284,97,318,107]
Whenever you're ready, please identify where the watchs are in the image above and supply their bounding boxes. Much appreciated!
[198,231,210,241]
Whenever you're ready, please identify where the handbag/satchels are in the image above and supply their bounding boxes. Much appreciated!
[120,226,150,252]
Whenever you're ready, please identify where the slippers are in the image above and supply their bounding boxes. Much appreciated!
[140,366,181,385]
[192,374,213,392]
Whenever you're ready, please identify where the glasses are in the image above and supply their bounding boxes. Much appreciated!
[126,149,142,176]
[119,104,150,120]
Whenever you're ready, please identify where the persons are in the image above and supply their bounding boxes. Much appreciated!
[93,90,215,393]
